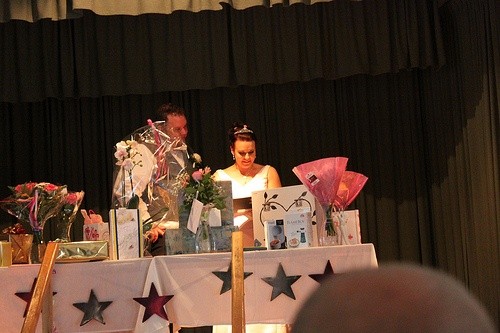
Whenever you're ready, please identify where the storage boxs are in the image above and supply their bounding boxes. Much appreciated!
[109,208,145,260]
[264,219,286,249]
[286,205,311,248]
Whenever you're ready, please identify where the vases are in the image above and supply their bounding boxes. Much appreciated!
[195,220,217,254]
[29,228,46,264]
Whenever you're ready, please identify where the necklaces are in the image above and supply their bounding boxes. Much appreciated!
[243,167,255,184]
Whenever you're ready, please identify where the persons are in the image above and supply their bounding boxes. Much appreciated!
[289,264,498,333]
[211,122,287,333]
[114,100,214,333]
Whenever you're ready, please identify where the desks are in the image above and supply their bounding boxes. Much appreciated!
[134,242,377,333]
[0,256,153,333]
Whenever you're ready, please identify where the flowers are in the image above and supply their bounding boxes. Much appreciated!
[183,166,228,240]
[1,181,66,242]
[52,189,85,240]
[114,139,143,209]
